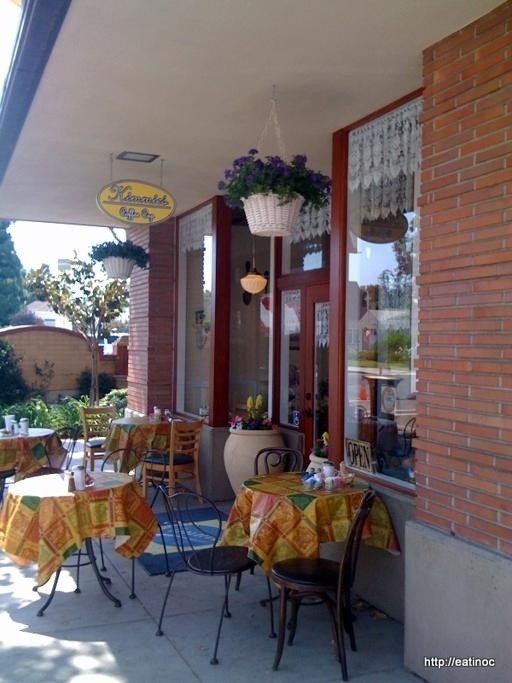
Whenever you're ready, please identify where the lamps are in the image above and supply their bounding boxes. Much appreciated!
[239,235,268,295]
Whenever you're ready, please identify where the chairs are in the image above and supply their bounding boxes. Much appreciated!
[377,416,416,479]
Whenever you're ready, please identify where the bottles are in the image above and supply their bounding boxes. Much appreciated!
[153,404,171,420]
[3,413,29,435]
[300,459,343,491]
[62,463,94,491]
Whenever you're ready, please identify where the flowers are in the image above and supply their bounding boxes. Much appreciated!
[89,240,152,268]
[217,148,334,214]
[228,394,280,432]
